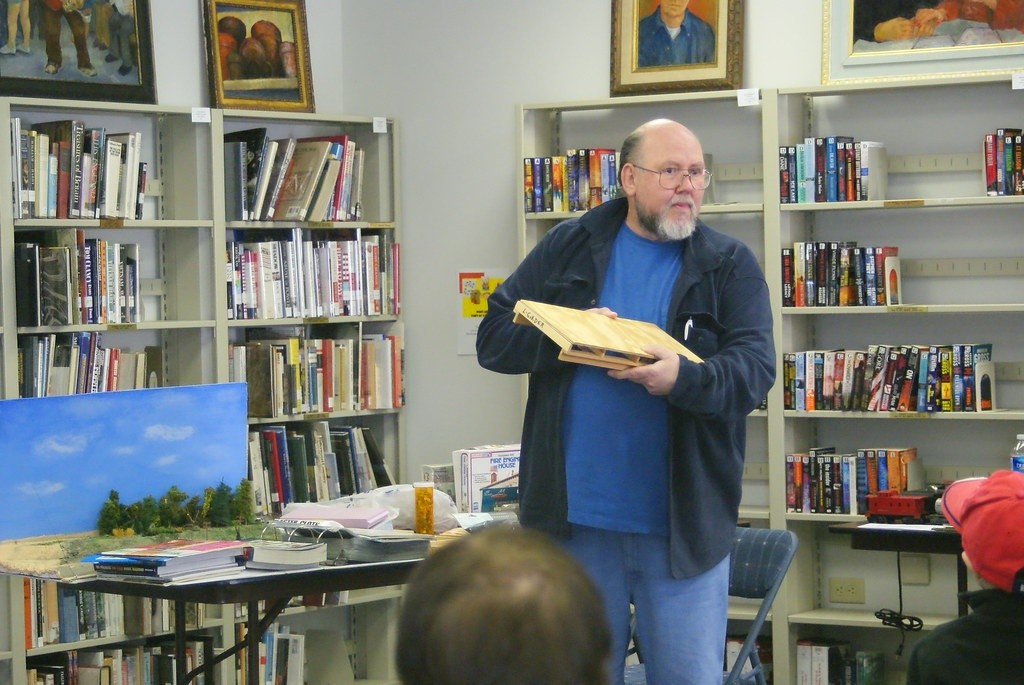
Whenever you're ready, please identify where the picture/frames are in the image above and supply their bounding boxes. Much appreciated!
[820,1,1024,86]
[201,0,316,113]
[609,0,745,99]
[0,0,159,105]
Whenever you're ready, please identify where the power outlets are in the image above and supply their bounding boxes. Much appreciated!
[829,577,866,604]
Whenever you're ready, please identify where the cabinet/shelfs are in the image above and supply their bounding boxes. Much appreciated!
[0,97,406,685]
[514,68,1024,685]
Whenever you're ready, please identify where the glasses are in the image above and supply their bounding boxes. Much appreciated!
[619,162,713,191]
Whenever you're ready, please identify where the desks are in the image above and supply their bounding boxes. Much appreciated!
[57,546,426,685]
[827,519,979,618]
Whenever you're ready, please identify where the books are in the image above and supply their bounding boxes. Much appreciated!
[258,523,436,563]
[783,343,998,412]
[92,538,327,586]
[779,135,888,204]
[13,228,140,326]
[10,117,147,220]
[20,575,352,684]
[780,240,902,306]
[723,633,886,685]
[248,420,396,516]
[524,146,623,213]
[784,446,918,516]
[18,330,149,398]
[225,227,400,320]
[982,128,1024,196]
[223,127,366,222]
[227,321,406,419]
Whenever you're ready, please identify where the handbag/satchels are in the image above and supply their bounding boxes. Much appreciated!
[324,484,460,532]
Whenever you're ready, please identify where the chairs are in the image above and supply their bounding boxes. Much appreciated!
[622,526,798,685]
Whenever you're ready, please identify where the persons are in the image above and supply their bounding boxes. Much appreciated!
[905,470,1024,685]
[477,117,777,685]
[394,526,615,685]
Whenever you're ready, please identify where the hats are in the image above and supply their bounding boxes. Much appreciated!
[943,470,1024,593]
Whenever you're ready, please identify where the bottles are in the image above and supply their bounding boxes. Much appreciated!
[1010,434,1024,473]
[412,482,434,535]
